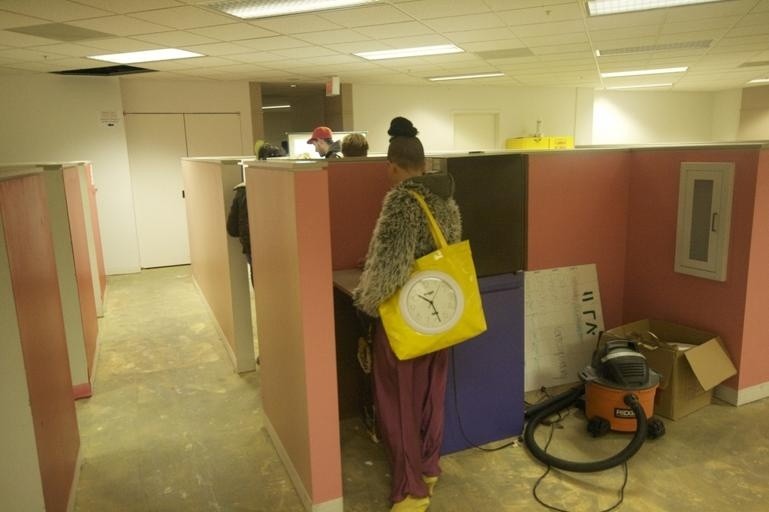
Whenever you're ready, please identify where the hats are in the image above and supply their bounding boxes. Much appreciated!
[306,126,332,144]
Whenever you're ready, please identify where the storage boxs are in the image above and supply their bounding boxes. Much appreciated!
[595,316,740,424]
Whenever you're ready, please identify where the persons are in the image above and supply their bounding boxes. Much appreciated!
[305,126,343,159]
[355,135,465,512]
[225,142,289,367]
[339,133,370,157]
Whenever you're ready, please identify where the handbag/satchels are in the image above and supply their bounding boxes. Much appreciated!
[376,189,488,361]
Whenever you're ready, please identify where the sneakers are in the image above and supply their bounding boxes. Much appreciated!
[391,490,431,512]
[421,474,440,494]
